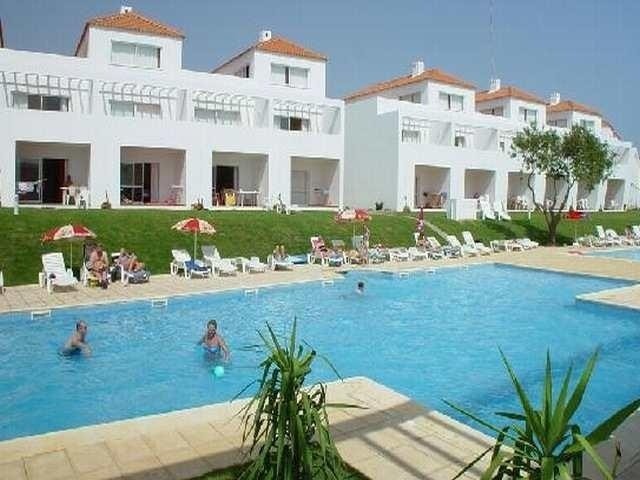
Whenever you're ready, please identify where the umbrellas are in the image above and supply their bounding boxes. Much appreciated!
[43,216,98,269]
[416,204,425,240]
[171,215,217,262]
[337,207,372,250]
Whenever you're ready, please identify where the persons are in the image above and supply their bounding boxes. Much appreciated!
[274,243,289,261]
[64,320,91,356]
[313,233,332,259]
[197,319,230,362]
[354,282,367,296]
[88,242,144,288]
[361,223,370,257]
[63,174,72,193]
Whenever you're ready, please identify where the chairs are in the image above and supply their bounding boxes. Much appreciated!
[569,225,639,250]
[511,195,619,210]
[34,227,153,294]
[167,231,540,286]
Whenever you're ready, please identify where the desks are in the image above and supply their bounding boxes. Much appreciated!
[59,184,261,208]
